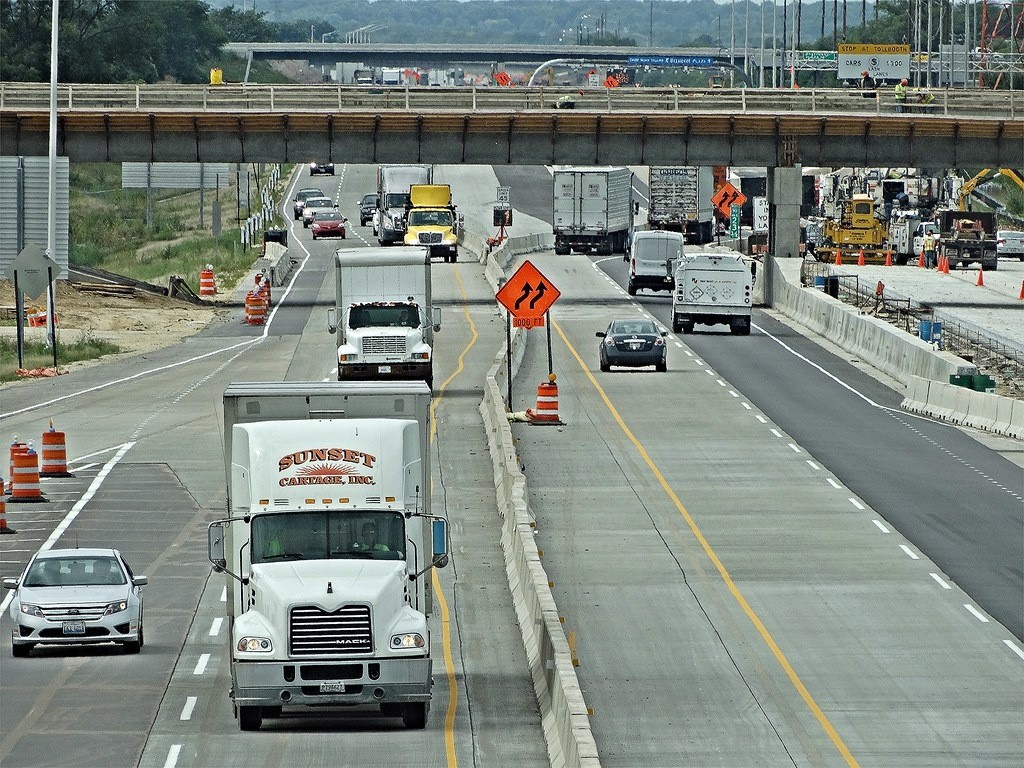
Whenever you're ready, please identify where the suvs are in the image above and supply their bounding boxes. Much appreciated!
[301,198,340,227]
[293,188,325,220]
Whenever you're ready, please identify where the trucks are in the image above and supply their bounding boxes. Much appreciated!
[375,162,435,247]
[204,379,450,732]
[401,183,461,263]
[623,229,686,294]
[670,251,757,335]
[327,244,442,393]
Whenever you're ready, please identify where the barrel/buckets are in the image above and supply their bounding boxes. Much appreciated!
[814,276,826,292]
[826,275,838,299]
[919,322,942,350]
[950,373,995,392]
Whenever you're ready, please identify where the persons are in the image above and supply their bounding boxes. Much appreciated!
[859,71,876,98]
[895,79,908,112]
[552,96,575,109]
[924,230,937,269]
[436,213,449,224]
[397,310,412,324]
[896,192,908,204]
[913,88,935,113]
[357,522,389,552]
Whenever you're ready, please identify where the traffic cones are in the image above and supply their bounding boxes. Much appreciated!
[1017,278,1024,300]
[936,253,944,271]
[858,249,866,267]
[885,249,893,266]
[917,250,926,268]
[835,247,842,266]
[942,257,951,274]
[974,266,986,287]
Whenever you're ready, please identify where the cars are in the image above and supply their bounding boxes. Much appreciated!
[718,223,728,236]
[997,230,1024,261]
[310,211,349,240]
[310,162,335,176]
[370,208,381,236]
[594,317,670,371]
[358,192,379,227]
[2,547,148,657]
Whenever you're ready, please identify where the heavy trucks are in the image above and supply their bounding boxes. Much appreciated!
[646,165,717,246]
[551,164,640,257]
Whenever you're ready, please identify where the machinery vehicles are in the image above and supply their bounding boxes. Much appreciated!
[807,165,1024,272]
